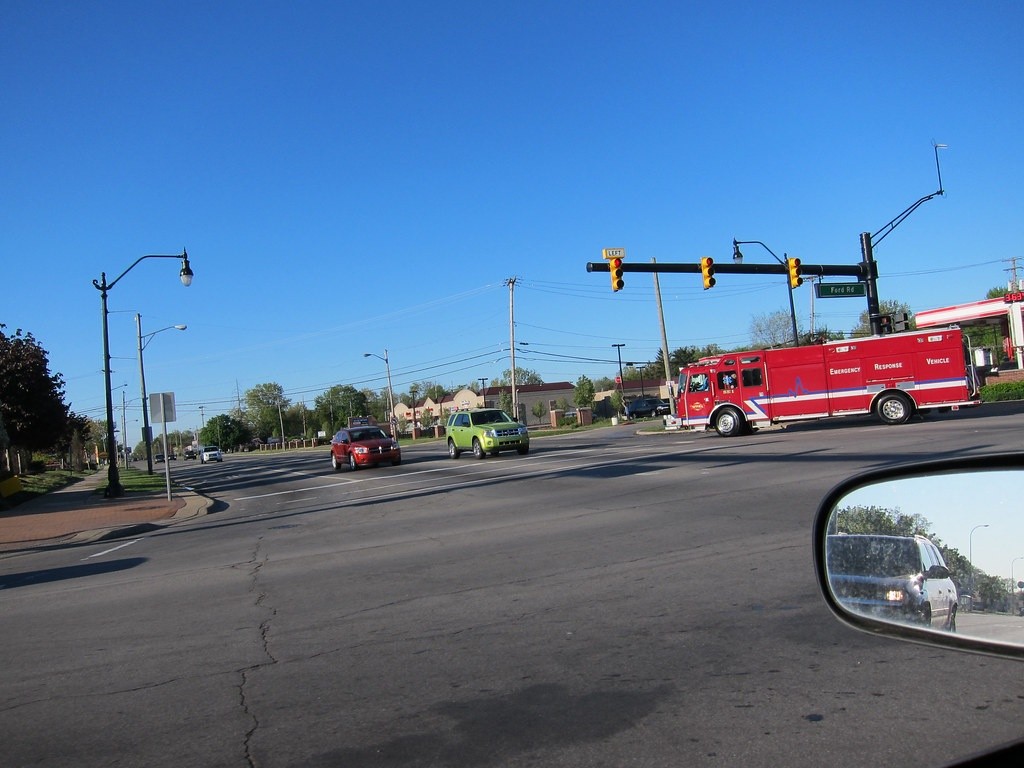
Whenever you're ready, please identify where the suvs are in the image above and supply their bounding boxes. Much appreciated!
[443,408,530,460]
[824,534,958,634]
[200,446,223,464]
[628,397,671,418]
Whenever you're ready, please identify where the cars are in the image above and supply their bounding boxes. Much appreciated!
[330,425,402,474]
[184,450,197,461]
[168,454,177,460]
[154,454,165,464]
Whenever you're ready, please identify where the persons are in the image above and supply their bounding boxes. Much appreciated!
[697,373,709,391]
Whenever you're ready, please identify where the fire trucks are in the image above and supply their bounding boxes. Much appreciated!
[667,324,982,439]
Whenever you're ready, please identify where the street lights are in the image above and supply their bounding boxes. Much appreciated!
[363,348,398,442]
[611,343,626,394]
[92,245,194,499]
[636,366,646,397]
[732,236,800,347]
[858,143,949,335]
[1011,557,1024,615]
[969,524,989,613]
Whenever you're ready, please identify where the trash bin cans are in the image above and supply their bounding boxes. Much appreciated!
[611,417,618,426]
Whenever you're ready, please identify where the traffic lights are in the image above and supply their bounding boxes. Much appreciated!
[788,257,803,288]
[701,256,716,290]
[610,257,625,291]
